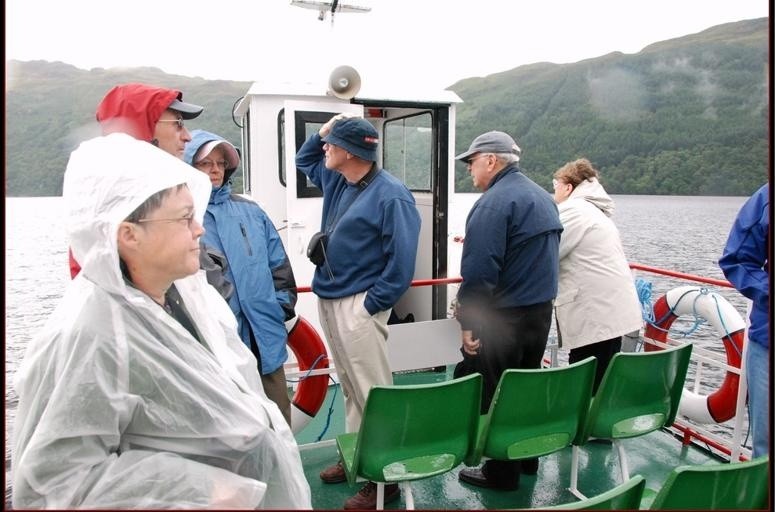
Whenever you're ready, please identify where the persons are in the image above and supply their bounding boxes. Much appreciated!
[68,82,235,305]
[295,112,421,511]
[718,184,770,462]
[10,131,312,510]
[554,153,643,446]
[180,129,298,429]
[455,129,563,492]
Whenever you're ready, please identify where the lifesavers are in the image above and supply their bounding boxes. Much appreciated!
[644,285,749,425]
[284,312,329,436]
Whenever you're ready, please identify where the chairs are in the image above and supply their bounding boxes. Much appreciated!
[648,452,770,510]
[509,474,645,510]
[336,374,482,510]
[565,342,693,501]
[463,357,597,467]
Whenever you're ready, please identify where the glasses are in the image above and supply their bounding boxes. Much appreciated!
[468,154,489,165]
[197,158,228,169]
[134,212,194,226]
[157,115,183,129]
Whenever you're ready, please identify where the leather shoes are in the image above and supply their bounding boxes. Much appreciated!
[458,468,521,491]
[319,462,347,483]
[344,482,400,510]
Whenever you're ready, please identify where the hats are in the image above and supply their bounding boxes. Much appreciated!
[194,139,240,171]
[167,100,203,119]
[322,117,379,162]
[454,132,520,161]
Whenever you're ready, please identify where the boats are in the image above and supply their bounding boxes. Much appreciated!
[218,0,771,511]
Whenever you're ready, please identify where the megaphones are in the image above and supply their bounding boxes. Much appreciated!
[326,66,361,100]
